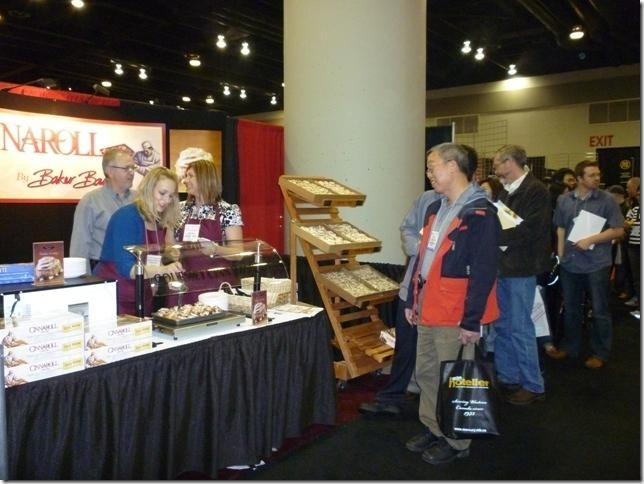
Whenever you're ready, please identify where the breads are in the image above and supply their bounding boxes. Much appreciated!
[157,302,222,321]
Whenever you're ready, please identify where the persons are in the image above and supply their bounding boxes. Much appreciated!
[172,158,246,261]
[67,148,138,272]
[475,143,640,405]
[90,165,182,317]
[132,140,159,175]
[404,140,499,465]
[357,149,449,414]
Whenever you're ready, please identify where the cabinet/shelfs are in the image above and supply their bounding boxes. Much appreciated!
[278,174,401,388]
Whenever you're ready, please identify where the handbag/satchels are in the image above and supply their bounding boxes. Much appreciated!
[436,342,501,440]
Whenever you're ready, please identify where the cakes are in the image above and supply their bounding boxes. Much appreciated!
[35,256,64,281]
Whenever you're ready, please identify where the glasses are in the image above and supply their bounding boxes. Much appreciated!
[110,165,137,173]
[428,159,452,174]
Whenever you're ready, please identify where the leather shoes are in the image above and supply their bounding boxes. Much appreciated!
[421,436,470,465]
[405,427,439,452]
[585,357,606,369]
[504,385,546,405]
[546,347,568,360]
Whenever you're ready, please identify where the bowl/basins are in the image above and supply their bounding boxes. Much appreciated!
[197,291,229,311]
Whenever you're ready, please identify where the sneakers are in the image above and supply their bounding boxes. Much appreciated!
[630,310,640,321]
[356,397,405,418]
[625,297,639,306]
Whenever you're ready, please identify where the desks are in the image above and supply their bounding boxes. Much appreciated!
[4,303,335,479]
